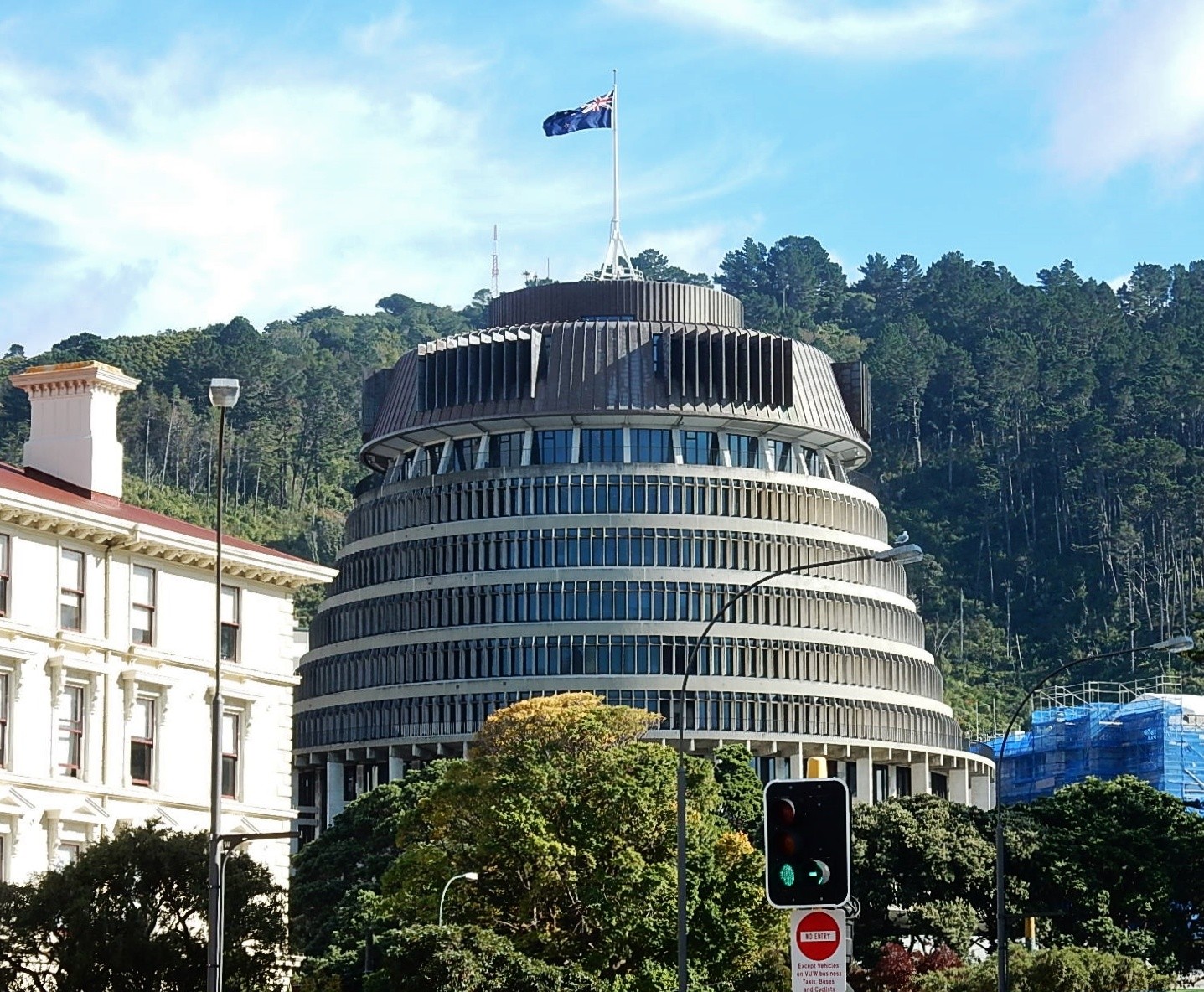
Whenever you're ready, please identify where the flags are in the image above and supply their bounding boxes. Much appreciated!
[542,88,615,136]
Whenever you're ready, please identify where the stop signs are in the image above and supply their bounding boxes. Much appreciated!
[795,912,840,962]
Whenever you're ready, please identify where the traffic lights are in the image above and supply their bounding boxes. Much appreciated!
[763,778,850,909]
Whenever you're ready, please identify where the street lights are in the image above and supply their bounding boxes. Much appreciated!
[678,545,924,992]
[994,635,1195,992]
[438,872,478,928]
[208,376,241,992]
